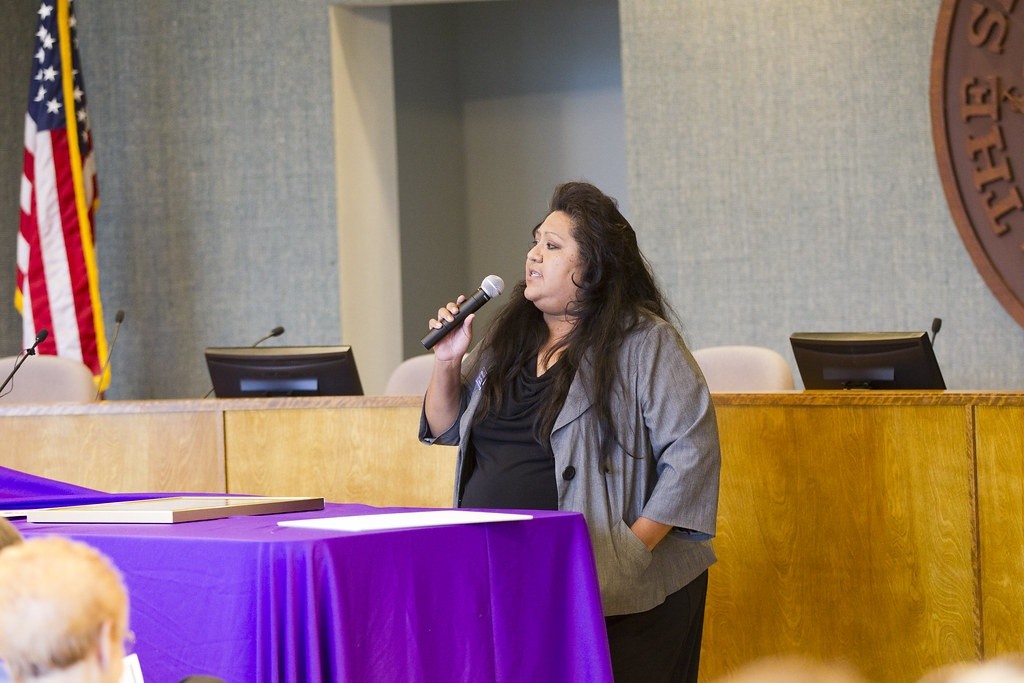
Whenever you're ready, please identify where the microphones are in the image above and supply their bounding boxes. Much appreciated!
[93,308,126,399]
[420,274,505,351]
[204,327,285,397]
[931,317,943,344]
[0,329,49,393]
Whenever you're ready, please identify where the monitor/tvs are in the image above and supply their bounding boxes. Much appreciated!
[789,331,946,390]
[206,345,366,397]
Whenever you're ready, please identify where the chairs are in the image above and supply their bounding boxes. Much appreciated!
[682,342,797,397]
[0,351,109,407]
[381,353,480,401]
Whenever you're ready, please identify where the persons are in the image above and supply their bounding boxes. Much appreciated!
[1,515,134,683]
[418,180,721,683]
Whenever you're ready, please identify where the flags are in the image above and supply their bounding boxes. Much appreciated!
[13,0,112,402]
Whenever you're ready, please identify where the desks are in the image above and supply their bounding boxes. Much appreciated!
[0,381,1024,683]
